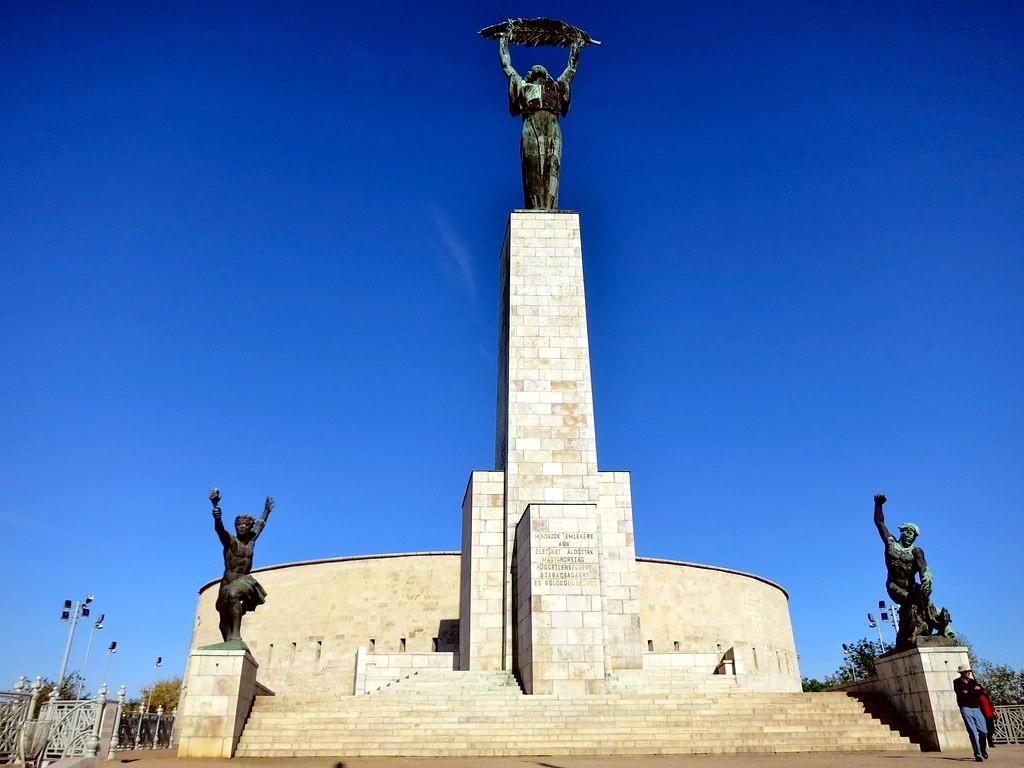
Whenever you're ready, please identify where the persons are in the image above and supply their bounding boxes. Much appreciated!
[211,488,275,640]
[954,664,989,762]
[978,689,999,748]
[498,32,581,211]
[872,493,932,644]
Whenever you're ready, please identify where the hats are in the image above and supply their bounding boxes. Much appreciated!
[957,664,973,673]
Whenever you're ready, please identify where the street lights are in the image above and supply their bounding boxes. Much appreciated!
[101,640,117,681]
[65,613,105,758]
[35,593,96,768]
[842,643,857,682]
[879,600,900,633]
[867,613,886,653]
[145,656,162,712]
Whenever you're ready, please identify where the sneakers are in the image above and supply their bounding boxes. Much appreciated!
[982,751,988,759]
[975,757,983,762]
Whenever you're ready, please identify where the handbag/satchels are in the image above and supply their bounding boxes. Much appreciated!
[993,709,1001,719]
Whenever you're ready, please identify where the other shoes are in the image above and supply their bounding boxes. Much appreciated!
[989,745,995,747]
